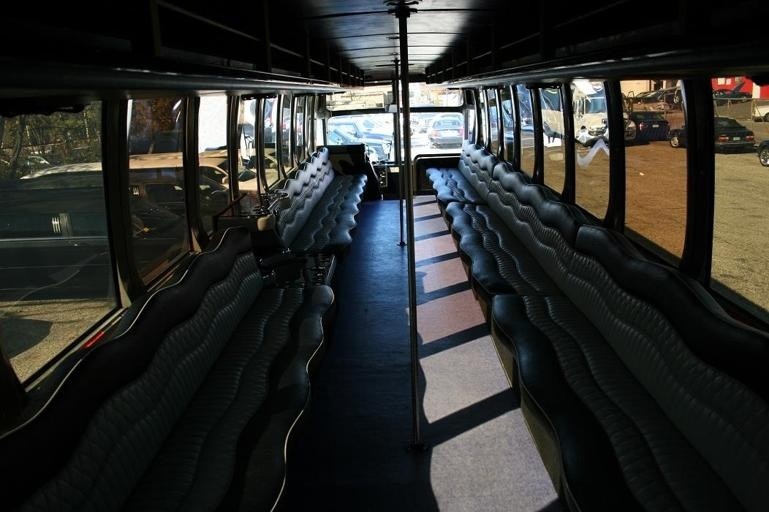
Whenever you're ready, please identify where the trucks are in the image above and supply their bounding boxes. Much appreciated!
[540,92,637,147]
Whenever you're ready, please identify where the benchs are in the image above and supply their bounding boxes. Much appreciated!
[426,134,768,510]
[0,144,370,511]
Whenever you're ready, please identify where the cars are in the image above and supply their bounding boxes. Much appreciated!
[753,104,769,122]
[630,111,669,140]
[635,89,753,109]
[757,141,769,166]
[666,117,755,151]
[414,98,463,146]
